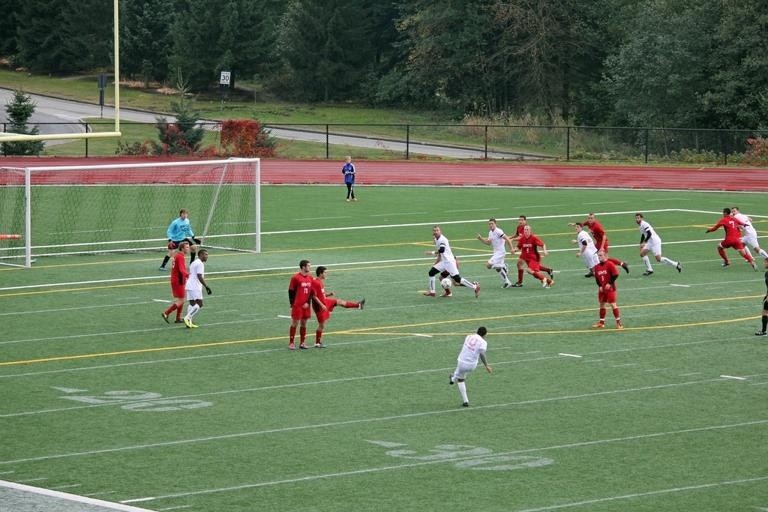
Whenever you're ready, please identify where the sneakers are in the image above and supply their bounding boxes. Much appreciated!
[542,267,555,289]
[590,321,605,329]
[643,269,655,275]
[448,373,456,385]
[622,260,632,274]
[298,343,309,350]
[615,320,624,330]
[474,281,481,299]
[289,342,297,351]
[314,341,327,349]
[500,281,524,289]
[754,330,767,336]
[183,317,198,328]
[745,257,760,272]
[719,261,731,267]
[159,265,168,271]
[423,287,453,297]
[461,402,469,408]
[160,312,185,325]
[676,261,682,274]
[345,198,358,202]
[358,298,366,310]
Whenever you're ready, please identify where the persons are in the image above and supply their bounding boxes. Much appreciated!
[342,156,357,202]
[755,258,768,336]
[159,209,201,269]
[449,328,492,407]
[635,213,682,275]
[288,260,314,349]
[162,241,189,324]
[477,215,554,287]
[312,266,365,348]
[183,250,212,329]
[593,249,623,329]
[568,213,629,277]
[706,207,767,268]
[424,226,480,296]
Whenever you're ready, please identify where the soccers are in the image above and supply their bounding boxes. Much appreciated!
[442,278,451,289]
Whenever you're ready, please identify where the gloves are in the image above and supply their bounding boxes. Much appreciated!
[192,237,201,244]
[167,241,175,249]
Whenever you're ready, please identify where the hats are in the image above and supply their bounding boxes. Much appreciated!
[344,155,352,160]
